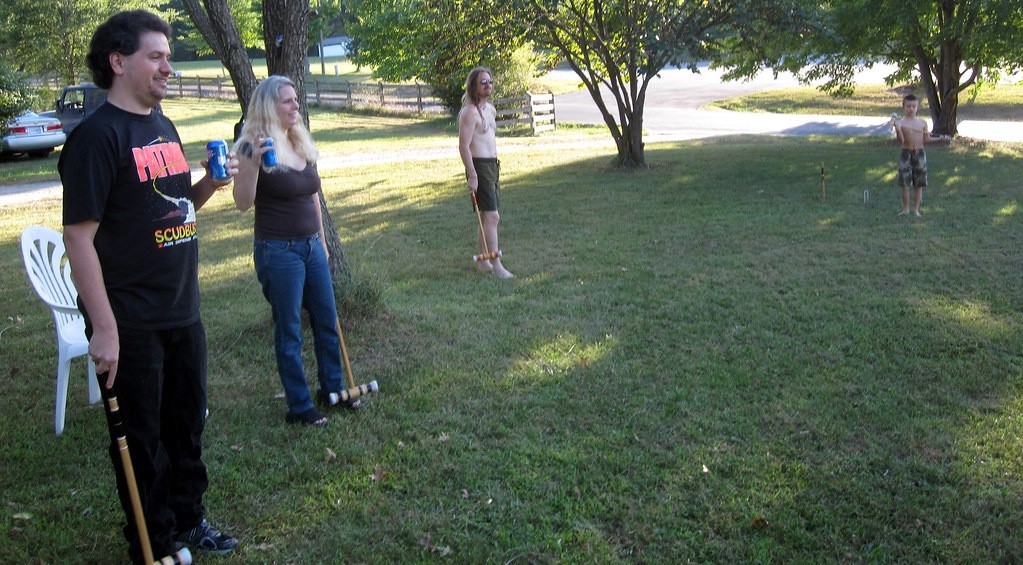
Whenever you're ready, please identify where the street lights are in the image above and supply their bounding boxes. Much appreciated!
[309,8,326,76]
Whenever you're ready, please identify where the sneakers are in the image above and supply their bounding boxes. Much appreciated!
[169,519,238,555]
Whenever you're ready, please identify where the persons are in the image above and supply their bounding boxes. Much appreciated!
[56,10,239,565]
[234,75,361,427]
[894,94,931,218]
[458,67,515,278]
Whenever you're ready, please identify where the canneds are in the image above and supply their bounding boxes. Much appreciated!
[207,140,232,183]
[259,137,278,167]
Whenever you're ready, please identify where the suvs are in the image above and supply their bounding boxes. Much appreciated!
[35,83,163,142]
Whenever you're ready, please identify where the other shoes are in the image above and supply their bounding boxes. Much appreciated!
[898,209,911,216]
[912,208,923,218]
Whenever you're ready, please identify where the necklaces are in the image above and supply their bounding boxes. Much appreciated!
[479,105,486,110]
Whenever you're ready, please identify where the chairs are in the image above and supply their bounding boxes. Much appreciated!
[21,226,209,437]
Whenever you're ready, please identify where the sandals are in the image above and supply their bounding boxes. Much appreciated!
[317,389,362,414]
[285,407,328,427]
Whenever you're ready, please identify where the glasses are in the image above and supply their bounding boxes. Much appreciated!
[480,79,493,84]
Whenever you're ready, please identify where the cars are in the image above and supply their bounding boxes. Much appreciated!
[0,109,67,162]
